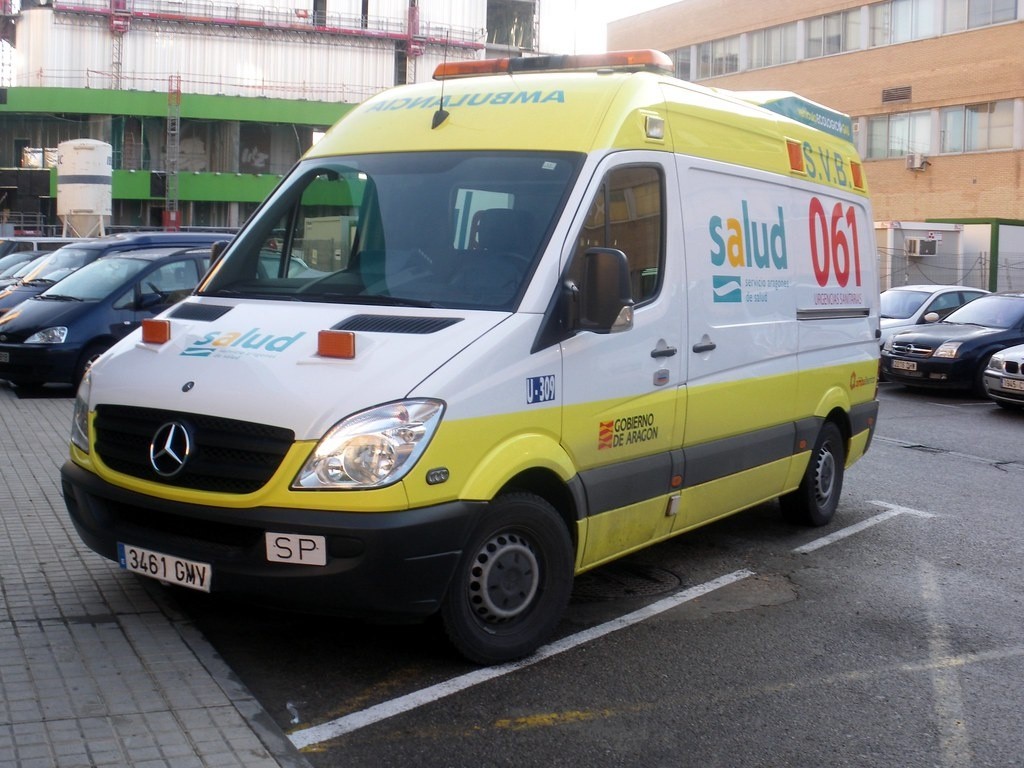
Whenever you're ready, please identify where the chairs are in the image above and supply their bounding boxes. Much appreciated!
[330,208,461,296]
[467,208,562,316]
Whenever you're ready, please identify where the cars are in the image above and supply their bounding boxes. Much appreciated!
[879,284,993,350]
[982,341,1024,411]
[0,218,345,397]
[880,289,1024,398]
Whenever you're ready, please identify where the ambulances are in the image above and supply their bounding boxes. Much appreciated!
[56,50,881,670]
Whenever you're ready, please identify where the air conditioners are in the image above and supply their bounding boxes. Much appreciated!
[905,154,926,171]
[905,237,939,256]
